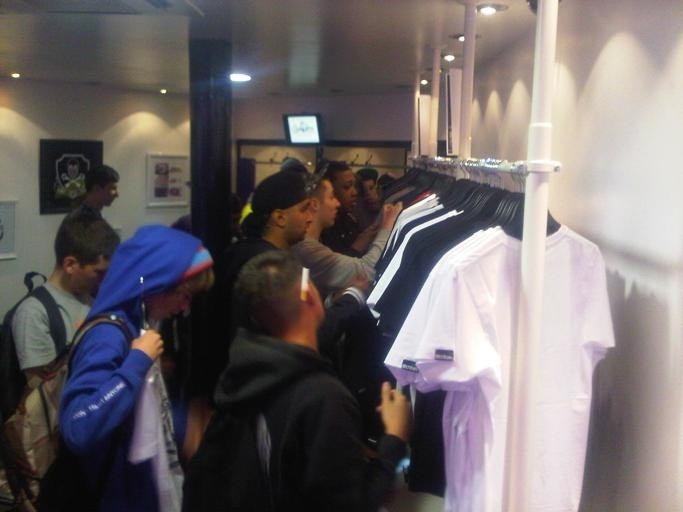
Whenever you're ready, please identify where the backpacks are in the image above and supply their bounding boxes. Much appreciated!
[0,272,134,479]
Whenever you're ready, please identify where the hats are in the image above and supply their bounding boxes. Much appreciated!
[355,168,377,180]
[251,158,330,209]
[178,245,213,282]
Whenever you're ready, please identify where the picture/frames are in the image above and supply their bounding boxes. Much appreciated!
[0,199,17,260]
[144,153,191,208]
[40,138,103,214]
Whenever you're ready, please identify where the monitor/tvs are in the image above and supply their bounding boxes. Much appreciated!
[282,113,322,146]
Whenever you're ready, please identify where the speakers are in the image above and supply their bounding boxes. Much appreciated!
[444,68,462,157]
[418,95,430,156]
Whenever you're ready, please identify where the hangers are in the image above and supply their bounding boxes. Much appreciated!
[398,147,560,237]
